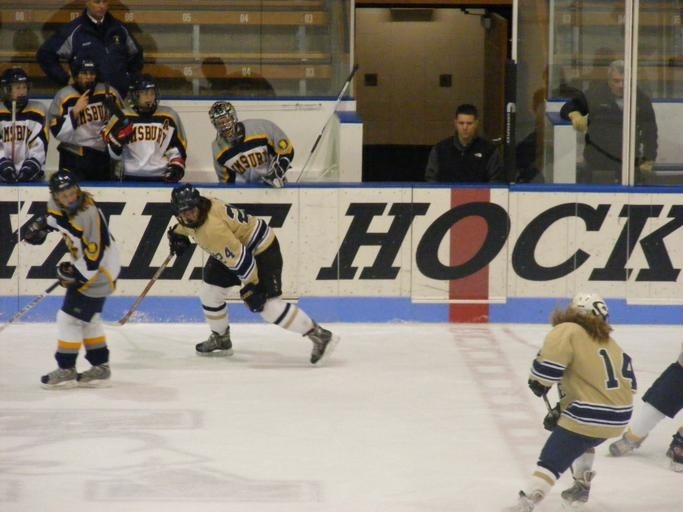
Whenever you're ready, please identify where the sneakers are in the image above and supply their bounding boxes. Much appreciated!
[41,368,77,384]
[77,365,111,382]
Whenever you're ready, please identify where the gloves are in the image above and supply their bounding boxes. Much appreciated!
[24,215,53,245]
[528,379,551,397]
[109,118,136,146]
[640,161,654,174]
[240,282,266,312]
[163,157,185,184]
[169,224,191,257]
[17,157,41,182]
[56,262,88,289]
[0,158,17,183]
[544,402,560,431]
[569,111,588,133]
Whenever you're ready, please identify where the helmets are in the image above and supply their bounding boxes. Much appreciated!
[208,101,239,142]
[49,168,83,213]
[570,292,608,322]
[129,73,160,115]
[171,183,204,227]
[70,55,98,96]
[0,67,30,115]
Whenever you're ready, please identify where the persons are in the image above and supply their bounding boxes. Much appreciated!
[558,59,658,185]
[424,104,504,185]
[608,346,683,466]
[34,1,144,97]
[209,100,295,185]
[519,291,637,512]
[165,187,333,366]
[22,169,123,386]
[1,59,187,183]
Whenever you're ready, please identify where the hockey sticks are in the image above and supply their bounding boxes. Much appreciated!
[101,253,174,328]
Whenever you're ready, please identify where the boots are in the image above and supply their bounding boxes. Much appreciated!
[561,471,596,503]
[667,431,683,464]
[609,431,648,456]
[506,491,543,512]
[196,326,232,352]
[302,321,331,363]
